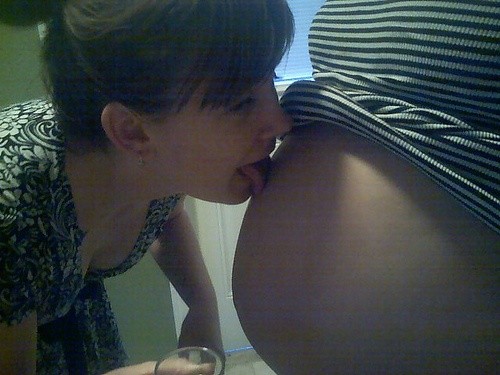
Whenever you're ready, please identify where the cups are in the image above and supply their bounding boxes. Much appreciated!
[155,345,225,375]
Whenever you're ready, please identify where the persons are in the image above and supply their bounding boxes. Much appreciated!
[0,0,291,375]
[232,0,500,375]
